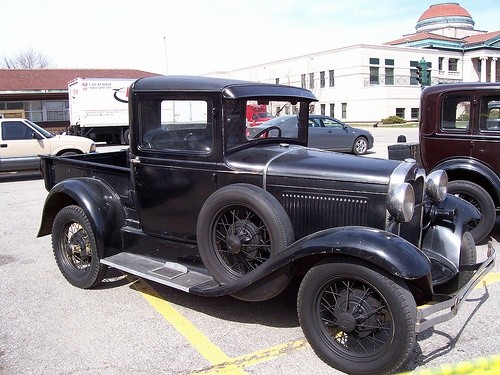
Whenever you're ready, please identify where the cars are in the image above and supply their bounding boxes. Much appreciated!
[388,82,500,246]
[246,114,374,155]
[0,117,98,180]
[35,75,497,375]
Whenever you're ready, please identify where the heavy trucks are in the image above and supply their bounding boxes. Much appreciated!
[67,77,274,141]
